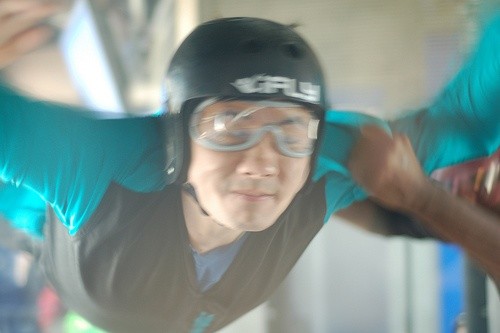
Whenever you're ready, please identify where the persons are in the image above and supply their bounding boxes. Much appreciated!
[333,119,500,294]
[1,0,500,333]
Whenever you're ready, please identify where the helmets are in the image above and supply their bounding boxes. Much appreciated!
[163,17,328,186]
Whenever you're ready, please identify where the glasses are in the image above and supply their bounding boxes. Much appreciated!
[189,96,319,156]
